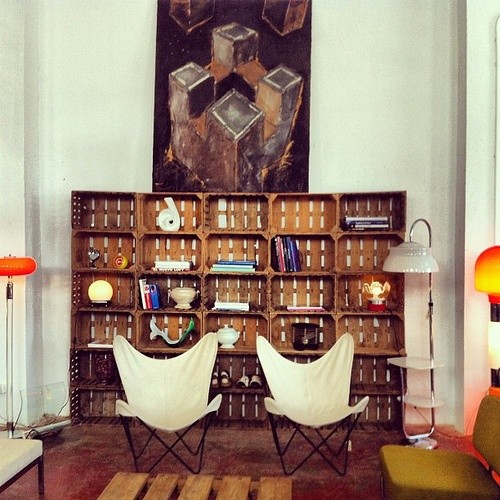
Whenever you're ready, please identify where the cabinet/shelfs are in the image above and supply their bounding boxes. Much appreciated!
[69,188,407,426]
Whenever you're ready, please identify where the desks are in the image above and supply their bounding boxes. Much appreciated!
[94,472,293,500]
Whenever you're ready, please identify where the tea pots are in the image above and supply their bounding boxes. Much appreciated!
[215,322,241,349]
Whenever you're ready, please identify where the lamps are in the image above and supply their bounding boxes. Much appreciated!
[473,245,500,396]
[382,218,445,449]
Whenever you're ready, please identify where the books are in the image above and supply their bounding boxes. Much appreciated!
[276,236,300,272]
[152,261,191,271]
[88,339,132,348]
[345,216,389,231]
[288,306,324,311]
[211,260,257,272]
[139,279,160,310]
[215,302,249,311]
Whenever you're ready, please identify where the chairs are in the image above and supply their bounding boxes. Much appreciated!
[112,332,222,474]
[256,333,369,476]
[380,395,500,500]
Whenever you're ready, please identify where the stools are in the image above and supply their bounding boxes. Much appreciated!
[0,439,44,500]
